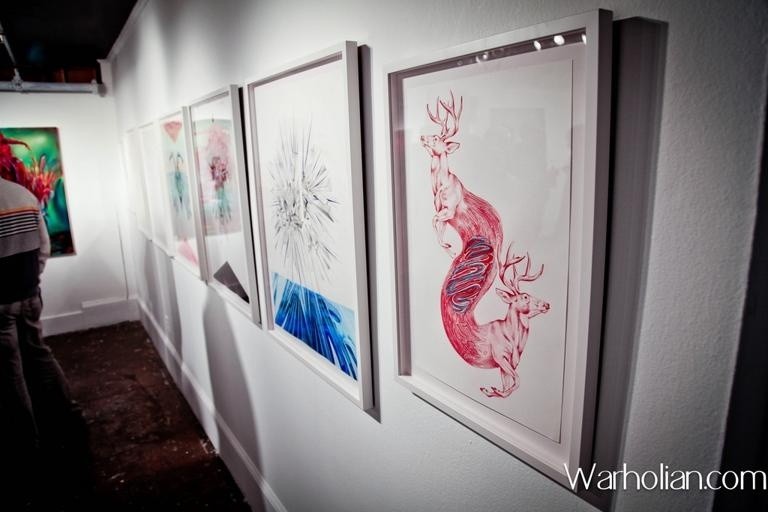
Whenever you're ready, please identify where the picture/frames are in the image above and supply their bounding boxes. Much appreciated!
[123,8,615,496]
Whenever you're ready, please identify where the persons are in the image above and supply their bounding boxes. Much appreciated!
[0,172,90,473]
